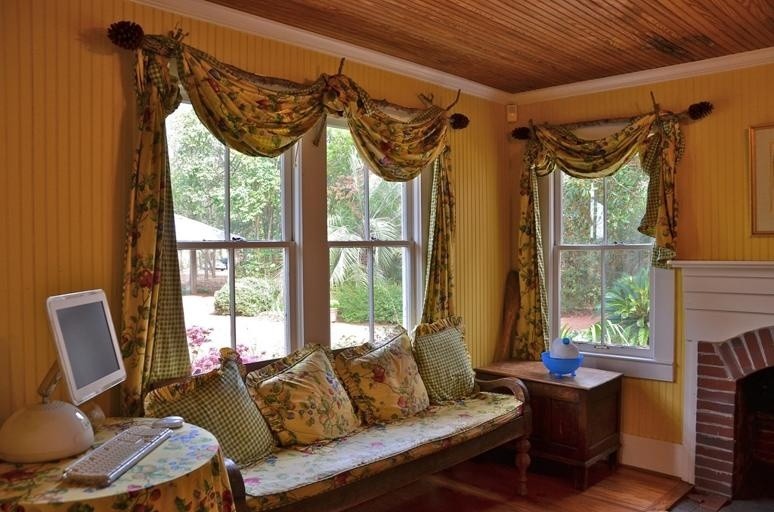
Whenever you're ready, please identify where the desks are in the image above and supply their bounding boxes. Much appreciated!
[0,413,222,512]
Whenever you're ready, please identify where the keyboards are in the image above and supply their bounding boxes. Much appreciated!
[65,425,172,488]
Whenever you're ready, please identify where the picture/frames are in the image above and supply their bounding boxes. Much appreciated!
[745,119,774,240]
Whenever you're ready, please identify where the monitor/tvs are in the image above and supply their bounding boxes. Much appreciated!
[0,290,127,465]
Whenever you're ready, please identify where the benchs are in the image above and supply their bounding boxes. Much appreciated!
[139,340,537,510]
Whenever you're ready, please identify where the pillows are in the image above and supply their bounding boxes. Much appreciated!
[335,322,439,428]
[408,313,482,407]
[139,345,279,474]
[243,339,368,455]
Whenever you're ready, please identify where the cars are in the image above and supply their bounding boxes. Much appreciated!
[201,259,226,271]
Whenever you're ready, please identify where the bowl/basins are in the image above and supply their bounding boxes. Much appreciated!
[540,350,585,379]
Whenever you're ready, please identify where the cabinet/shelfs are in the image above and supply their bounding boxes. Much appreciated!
[470,356,627,492]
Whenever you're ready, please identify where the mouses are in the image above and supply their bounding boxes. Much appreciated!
[152,416,183,429]
